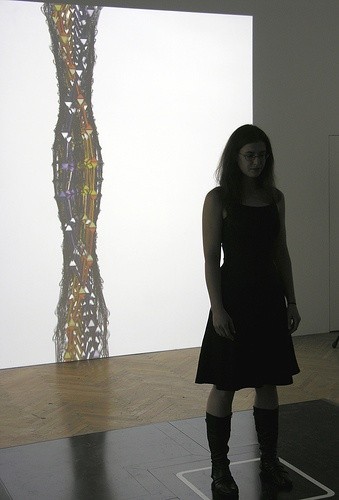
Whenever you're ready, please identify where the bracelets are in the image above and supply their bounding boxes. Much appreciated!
[288,302,296,305]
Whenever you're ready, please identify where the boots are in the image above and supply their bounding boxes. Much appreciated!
[206,411,239,496]
[253,405,293,488]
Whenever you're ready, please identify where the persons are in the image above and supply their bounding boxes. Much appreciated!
[195,124,301,497]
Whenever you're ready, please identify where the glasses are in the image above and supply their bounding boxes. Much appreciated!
[239,152,269,160]
